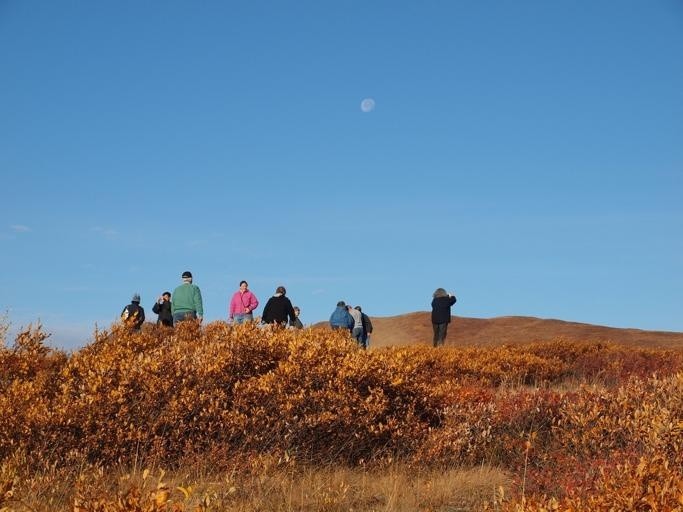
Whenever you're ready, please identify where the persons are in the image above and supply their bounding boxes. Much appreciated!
[226,281,258,324]
[287,306,304,329]
[260,286,295,328]
[354,306,373,349]
[151,292,172,328]
[170,271,203,328]
[329,301,353,333]
[119,295,144,333]
[430,288,456,348]
[345,306,362,345]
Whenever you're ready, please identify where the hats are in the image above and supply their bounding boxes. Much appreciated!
[132,296,140,303]
[182,272,192,278]
